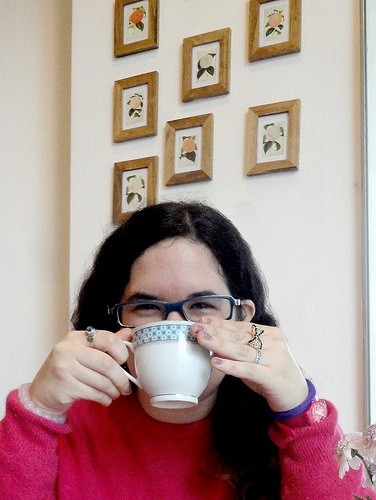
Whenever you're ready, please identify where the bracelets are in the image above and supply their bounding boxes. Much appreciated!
[270,378,316,420]
[17,382,67,424]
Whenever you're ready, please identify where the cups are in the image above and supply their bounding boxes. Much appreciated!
[119,320,212,409]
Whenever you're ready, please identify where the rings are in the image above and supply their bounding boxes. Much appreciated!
[248,323,265,349]
[253,348,262,363]
[85,326,96,349]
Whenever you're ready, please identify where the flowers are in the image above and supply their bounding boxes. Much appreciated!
[333,422,376,489]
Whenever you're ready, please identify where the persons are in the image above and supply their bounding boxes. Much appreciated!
[0,200,376,500]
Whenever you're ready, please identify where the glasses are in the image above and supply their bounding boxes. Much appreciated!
[104,295,244,328]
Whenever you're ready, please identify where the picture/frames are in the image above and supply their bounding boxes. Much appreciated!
[111,71,159,143]
[248,0,302,63]
[113,0,159,59]
[182,26,232,104]
[112,155,160,226]
[163,113,214,187]
[244,99,301,177]
